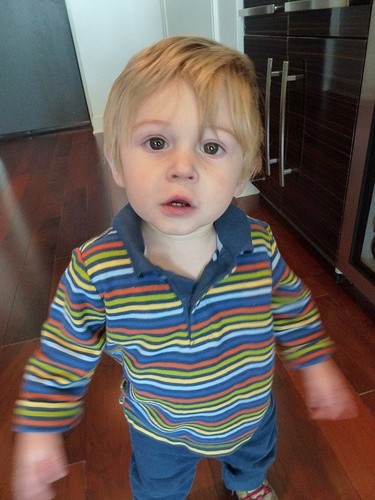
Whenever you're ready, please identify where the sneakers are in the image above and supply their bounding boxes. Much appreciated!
[236,479,278,500]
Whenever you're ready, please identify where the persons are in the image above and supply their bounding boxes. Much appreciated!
[10,36,361,500]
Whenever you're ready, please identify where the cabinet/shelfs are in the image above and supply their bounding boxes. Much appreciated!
[235,0,375,284]
